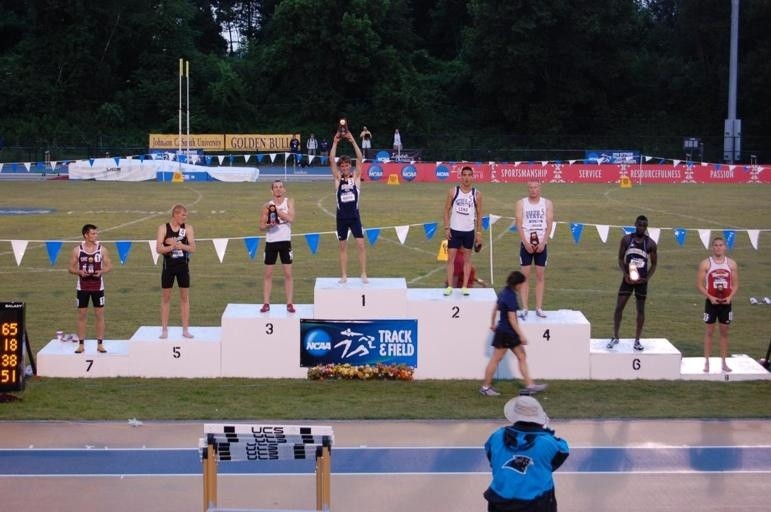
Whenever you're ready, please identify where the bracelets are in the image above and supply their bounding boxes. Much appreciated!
[445,223,448,229]
[478,279,482,284]
[476,232,481,234]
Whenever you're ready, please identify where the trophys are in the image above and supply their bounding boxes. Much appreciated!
[530,232,539,246]
[474,242,481,252]
[85,256,96,275]
[163,235,172,256]
[339,118,349,138]
[716,283,727,303]
[266,205,281,224]
[628,261,641,282]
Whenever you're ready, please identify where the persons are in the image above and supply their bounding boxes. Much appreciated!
[602,215,658,350]
[483,396,570,512]
[289,125,402,167]
[477,270,549,396]
[156,205,196,338]
[697,238,739,372]
[515,180,553,317]
[445,243,488,288]
[330,130,370,284]
[443,167,481,296]
[259,179,297,313]
[68,225,112,353]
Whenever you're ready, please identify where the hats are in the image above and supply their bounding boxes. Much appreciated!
[503,395,547,425]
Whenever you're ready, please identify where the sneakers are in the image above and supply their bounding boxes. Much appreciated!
[461,287,469,295]
[287,304,295,313]
[361,273,369,284]
[704,362,709,372]
[633,340,644,350]
[97,346,107,353]
[480,386,501,397]
[536,309,546,317]
[338,277,347,284]
[75,347,84,354]
[527,384,546,395]
[444,286,453,296]
[520,310,528,318]
[722,365,732,372]
[607,337,619,348]
[260,304,270,313]
